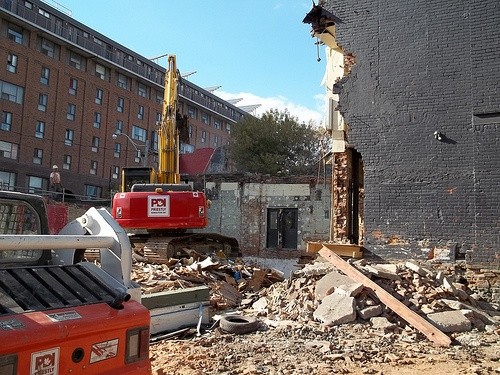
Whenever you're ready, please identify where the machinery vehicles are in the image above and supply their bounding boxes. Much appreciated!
[83,55,240,265]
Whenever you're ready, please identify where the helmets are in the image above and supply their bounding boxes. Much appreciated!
[53,165,58,168]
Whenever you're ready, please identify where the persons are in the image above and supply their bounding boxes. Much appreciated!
[50,165,62,202]
